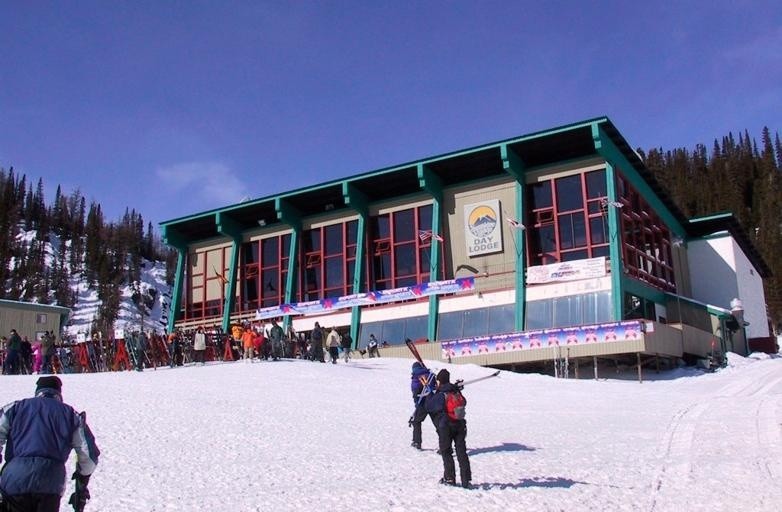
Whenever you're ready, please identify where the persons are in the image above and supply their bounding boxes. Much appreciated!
[422,367,472,489]
[167,326,209,368]
[1,329,53,374]
[366,333,381,358]
[410,360,443,455]
[300,322,353,364]
[0,376,100,512]
[52,330,151,374]
[234,320,286,362]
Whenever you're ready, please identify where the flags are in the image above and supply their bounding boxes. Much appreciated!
[504,215,526,231]
[214,270,229,283]
[418,229,444,243]
[598,195,625,209]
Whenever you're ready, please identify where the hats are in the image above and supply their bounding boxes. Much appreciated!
[411,362,420,367]
[437,369,449,383]
[35,376,63,392]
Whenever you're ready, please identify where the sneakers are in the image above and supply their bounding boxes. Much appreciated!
[412,442,422,449]
[440,477,456,485]
[463,482,469,488]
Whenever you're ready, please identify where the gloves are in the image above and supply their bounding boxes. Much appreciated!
[69,471,91,512]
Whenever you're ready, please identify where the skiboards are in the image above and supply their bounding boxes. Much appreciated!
[418,370,500,398]
[86,325,223,372]
[405,337,425,367]
